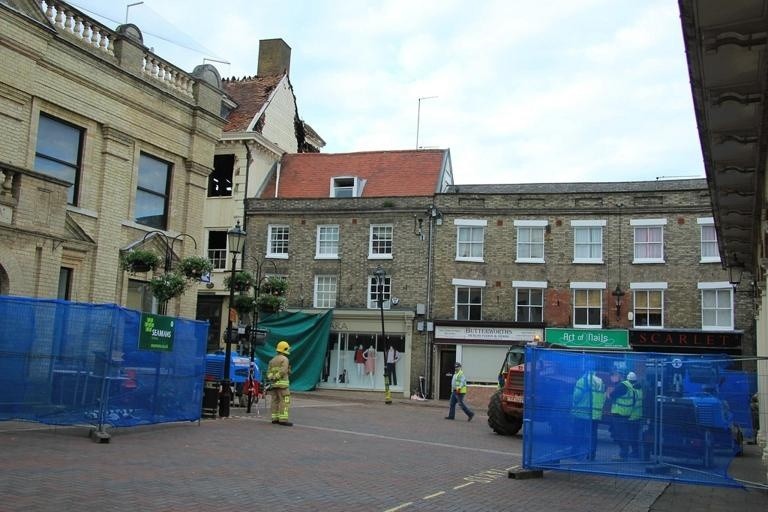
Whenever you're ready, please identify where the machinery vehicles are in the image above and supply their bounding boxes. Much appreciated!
[488,339,643,439]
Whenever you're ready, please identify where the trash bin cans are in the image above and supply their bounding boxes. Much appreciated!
[200,382,221,420]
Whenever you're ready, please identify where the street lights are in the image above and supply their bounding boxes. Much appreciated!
[220,219,248,417]
[372,264,394,404]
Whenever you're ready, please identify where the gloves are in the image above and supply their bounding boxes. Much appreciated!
[455,388,460,395]
[268,375,278,383]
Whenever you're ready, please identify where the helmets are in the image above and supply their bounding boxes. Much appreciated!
[627,371,637,381]
[276,341,292,355]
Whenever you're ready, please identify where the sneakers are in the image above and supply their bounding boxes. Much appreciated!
[272,420,279,424]
[445,416,455,420]
[570,450,641,462]
[279,422,293,426]
[468,413,475,422]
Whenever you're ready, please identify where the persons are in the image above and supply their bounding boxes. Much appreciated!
[263,340,292,427]
[569,365,606,462]
[353,344,365,386]
[386,345,400,386]
[362,345,376,386]
[608,365,636,463]
[624,371,643,459]
[443,362,475,422]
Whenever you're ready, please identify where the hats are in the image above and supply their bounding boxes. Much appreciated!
[615,366,629,376]
[454,362,462,368]
